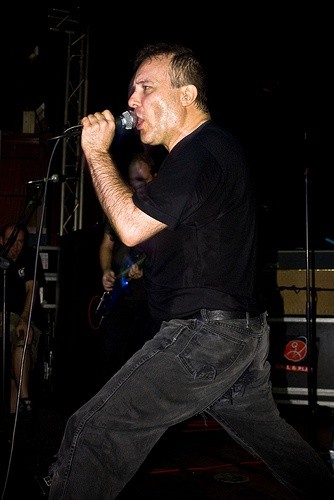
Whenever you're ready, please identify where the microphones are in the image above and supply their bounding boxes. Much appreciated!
[26,174,60,185]
[62,110,138,137]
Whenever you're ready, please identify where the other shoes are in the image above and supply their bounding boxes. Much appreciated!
[19,400,32,417]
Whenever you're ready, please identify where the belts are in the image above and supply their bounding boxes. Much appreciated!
[183,310,261,320]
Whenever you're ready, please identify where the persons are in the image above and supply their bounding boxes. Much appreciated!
[101,159,155,379]
[50,44,334,500]
[0,224,39,413]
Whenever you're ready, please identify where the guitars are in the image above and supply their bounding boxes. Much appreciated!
[87,256,150,331]
